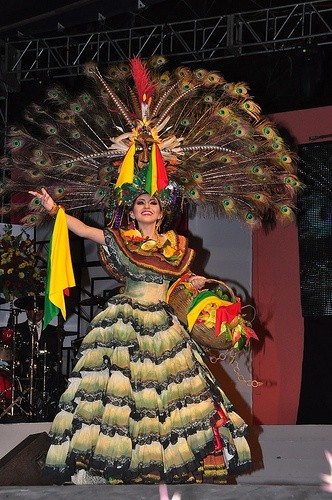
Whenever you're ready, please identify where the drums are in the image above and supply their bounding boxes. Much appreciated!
[0,366,24,415]
[71,336,84,360]
[0,326,25,361]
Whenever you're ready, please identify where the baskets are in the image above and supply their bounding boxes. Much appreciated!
[169,278,237,350]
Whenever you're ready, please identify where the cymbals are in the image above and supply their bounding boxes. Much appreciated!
[81,295,113,307]
[0,297,7,305]
[13,294,46,310]
[43,331,79,339]
[0,308,25,313]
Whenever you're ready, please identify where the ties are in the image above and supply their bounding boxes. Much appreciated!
[31,323,38,350]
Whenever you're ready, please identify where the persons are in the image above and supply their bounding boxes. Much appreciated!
[15,309,59,365]
[29,192,253,486]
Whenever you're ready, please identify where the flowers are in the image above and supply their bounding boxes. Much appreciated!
[0,223,47,304]
